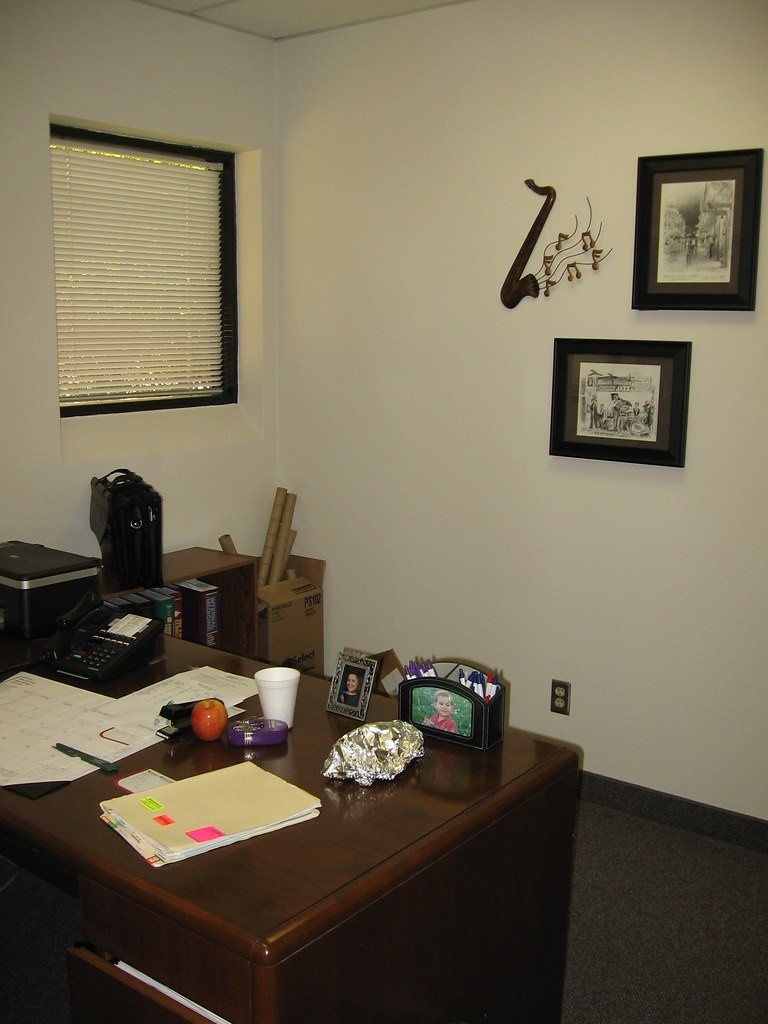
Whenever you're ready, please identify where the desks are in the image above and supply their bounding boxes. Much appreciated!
[0,547,579,1024]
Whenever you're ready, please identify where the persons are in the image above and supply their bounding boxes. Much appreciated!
[337,671,360,707]
[422,690,458,733]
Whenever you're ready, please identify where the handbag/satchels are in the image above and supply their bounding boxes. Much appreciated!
[89,468,165,591]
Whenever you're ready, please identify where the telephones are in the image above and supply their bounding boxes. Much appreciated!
[43,591,165,684]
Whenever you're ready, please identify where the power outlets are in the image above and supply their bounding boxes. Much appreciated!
[551,679,571,715]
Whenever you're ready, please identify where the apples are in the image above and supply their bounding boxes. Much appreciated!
[191,699,228,741]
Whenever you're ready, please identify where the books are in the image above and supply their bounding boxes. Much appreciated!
[99,761,322,869]
[103,578,220,649]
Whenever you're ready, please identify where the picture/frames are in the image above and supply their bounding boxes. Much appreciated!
[325,650,378,721]
[632,148,765,311]
[549,338,693,467]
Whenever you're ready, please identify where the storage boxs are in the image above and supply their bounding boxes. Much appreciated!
[329,647,406,699]
[257,555,327,678]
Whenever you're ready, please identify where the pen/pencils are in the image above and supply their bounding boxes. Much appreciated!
[400,655,439,681]
[457,666,501,701]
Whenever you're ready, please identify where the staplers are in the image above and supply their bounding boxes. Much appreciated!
[155,697,225,741]
